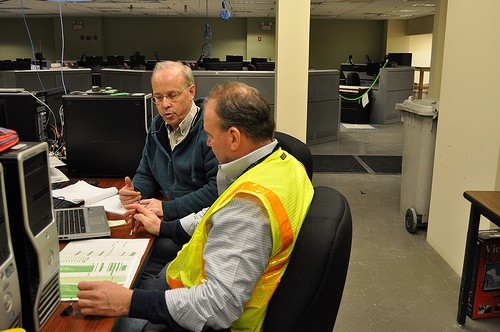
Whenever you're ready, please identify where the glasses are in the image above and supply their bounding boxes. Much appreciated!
[150,85,189,104]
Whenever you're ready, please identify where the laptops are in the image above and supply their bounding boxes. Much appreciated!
[53,205,112,242]
[49,180,98,209]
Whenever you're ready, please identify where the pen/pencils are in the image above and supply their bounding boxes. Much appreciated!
[130,209,138,235]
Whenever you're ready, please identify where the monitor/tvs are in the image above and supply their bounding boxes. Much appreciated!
[85,55,146,68]
[366,62,380,76]
[388,52,411,66]
[202,55,267,69]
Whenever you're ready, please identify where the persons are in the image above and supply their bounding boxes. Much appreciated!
[77,79,315,332]
[118,61,220,263]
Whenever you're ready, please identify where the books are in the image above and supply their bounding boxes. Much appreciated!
[51,180,127,216]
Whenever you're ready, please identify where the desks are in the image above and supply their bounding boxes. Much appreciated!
[457,191,500,325]
[37,176,166,332]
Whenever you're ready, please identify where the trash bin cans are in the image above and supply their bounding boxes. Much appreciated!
[395,95,439,234]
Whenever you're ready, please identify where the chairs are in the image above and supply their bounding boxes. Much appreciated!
[338,68,360,85]
[0,52,275,73]
[263,185,353,332]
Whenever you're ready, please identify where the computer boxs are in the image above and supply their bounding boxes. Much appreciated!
[0,140,65,332]
[0,91,48,141]
[62,93,155,179]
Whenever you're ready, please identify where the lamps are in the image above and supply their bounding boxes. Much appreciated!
[261,21,272,30]
[72,20,83,30]
